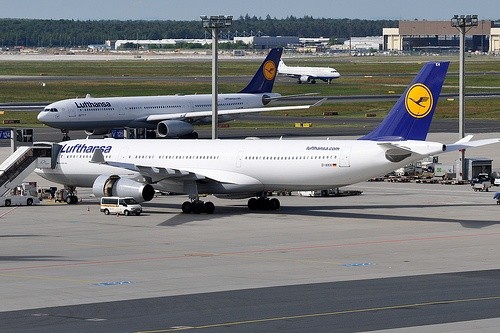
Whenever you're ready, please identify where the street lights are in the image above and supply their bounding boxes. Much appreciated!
[450,14,478,181]
[200,14,233,140]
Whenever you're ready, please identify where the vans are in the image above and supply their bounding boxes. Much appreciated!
[100,196,142,216]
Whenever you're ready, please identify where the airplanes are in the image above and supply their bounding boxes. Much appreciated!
[0,62,500,216]
[37,47,327,141]
[277,57,341,84]
